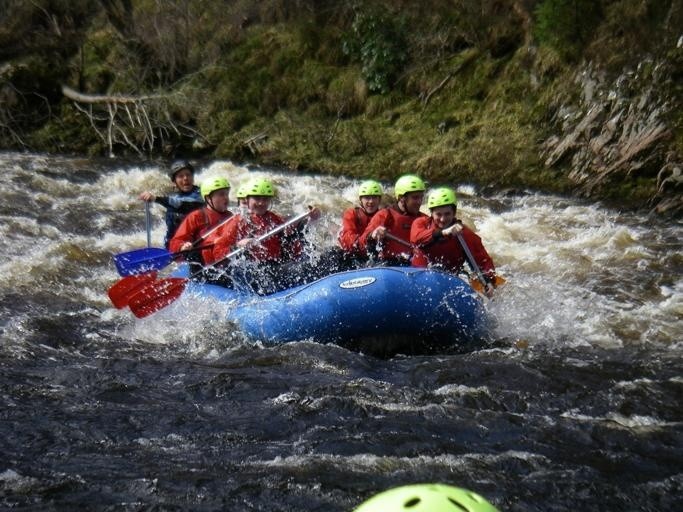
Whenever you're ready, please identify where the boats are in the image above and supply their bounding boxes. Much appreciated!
[158,261,488,354]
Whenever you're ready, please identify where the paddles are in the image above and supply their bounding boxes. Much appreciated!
[386,231,505,294]
[113,242,214,277]
[108,211,240,309]
[128,210,312,318]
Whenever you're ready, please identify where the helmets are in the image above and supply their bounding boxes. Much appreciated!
[236,182,249,198]
[394,174,426,202]
[358,181,383,197]
[169,160,194,182]
[428,186,458,210]
[200,175,231,203]
[244,178,275,197]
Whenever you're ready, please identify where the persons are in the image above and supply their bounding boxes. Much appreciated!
[137,159,207,250]
[338,179,383,257]
[212,178,321,276]
[410,186,496,300]
[200,184,247,267]
[359,174,430,267]
[169,175,234,274]
